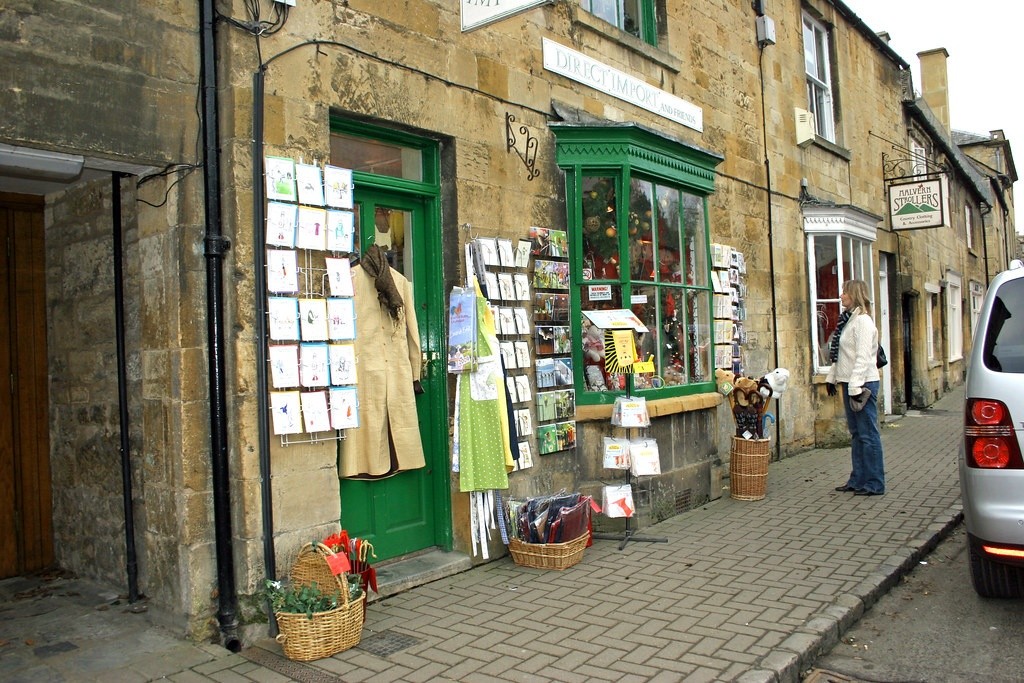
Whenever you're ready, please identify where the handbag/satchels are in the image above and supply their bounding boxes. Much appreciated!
[877,343,888,368]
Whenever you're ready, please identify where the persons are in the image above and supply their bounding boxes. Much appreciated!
[824,280,889,497]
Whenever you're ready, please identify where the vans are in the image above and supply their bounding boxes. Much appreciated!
[959,259,1024,597]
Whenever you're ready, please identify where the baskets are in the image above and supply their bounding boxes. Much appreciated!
[272,542,365,662]
[729,435,771,501]
[506,526,591,570]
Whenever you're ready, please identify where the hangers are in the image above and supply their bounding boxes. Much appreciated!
[368,236,378,249]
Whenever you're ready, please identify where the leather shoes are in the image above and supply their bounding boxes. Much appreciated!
[835,485,854,492]
[855,487,884,495]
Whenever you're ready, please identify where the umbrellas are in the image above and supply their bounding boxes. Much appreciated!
[732,383,774,439]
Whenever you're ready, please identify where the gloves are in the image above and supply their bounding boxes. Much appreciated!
[826,382,837,396]
[851,391,863,403]
